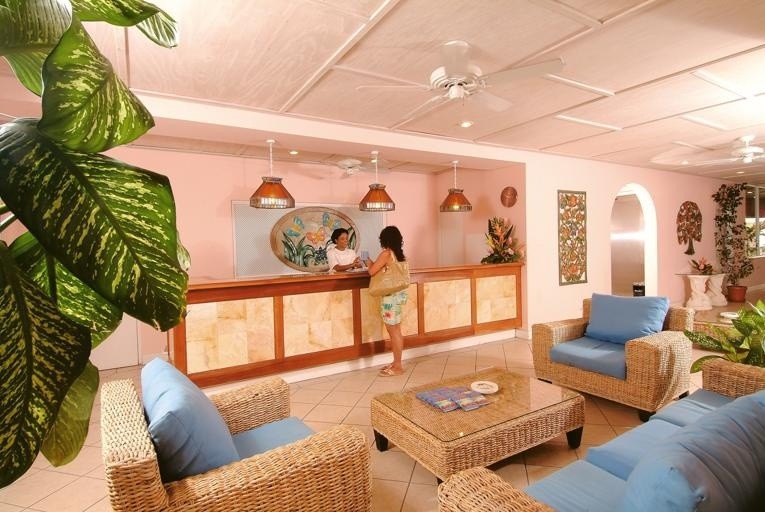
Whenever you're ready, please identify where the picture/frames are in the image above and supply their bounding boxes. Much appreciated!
[231,198,389,282]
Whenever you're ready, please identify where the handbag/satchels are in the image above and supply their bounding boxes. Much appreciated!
[369,262,409,297]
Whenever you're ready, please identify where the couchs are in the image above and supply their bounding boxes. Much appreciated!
[531,299,696,422]
[98,377,373,512]
[436,360,764,512]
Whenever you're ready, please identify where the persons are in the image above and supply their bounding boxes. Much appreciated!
[363,226,409,378]
[324,228,363,274]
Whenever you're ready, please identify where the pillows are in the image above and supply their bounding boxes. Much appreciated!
[140,357,241,477]
[622,388,764,512]
[584,292,670,345]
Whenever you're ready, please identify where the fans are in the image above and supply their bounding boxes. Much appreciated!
[671,136,764,171]
[330,37,565,133]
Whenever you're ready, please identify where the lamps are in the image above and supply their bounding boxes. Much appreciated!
[249,138,296,210]
[357,151,397,212]
[439,160,472,213]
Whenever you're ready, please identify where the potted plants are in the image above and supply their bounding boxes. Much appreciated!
[711,180,758,304]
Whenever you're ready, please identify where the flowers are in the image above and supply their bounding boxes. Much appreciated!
[691,256,714,274]
[480,216,525,263]
